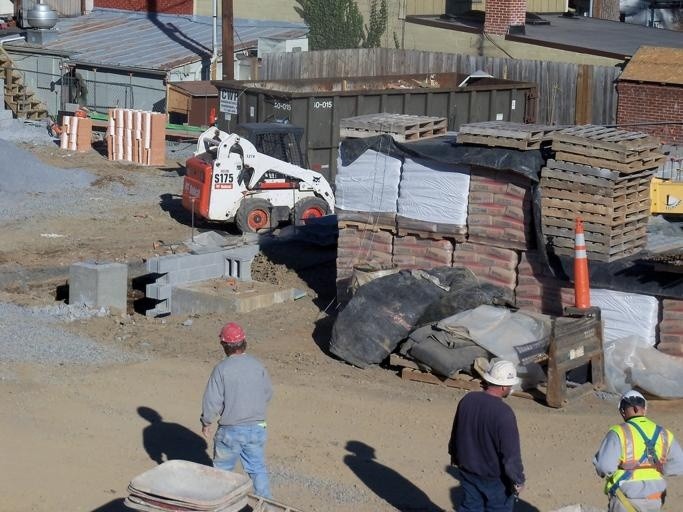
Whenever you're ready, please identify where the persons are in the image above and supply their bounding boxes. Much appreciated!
[446,359,526,512]
[591,389,683,512]
[200,321,278,512]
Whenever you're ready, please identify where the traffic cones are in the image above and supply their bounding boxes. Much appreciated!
[565,218,600,316]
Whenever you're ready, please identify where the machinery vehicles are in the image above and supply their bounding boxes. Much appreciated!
[183,122,335,234]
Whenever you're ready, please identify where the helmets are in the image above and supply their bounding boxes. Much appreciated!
[478,360,521,386]
[616,390,648,412]
[216,321,247,344]
